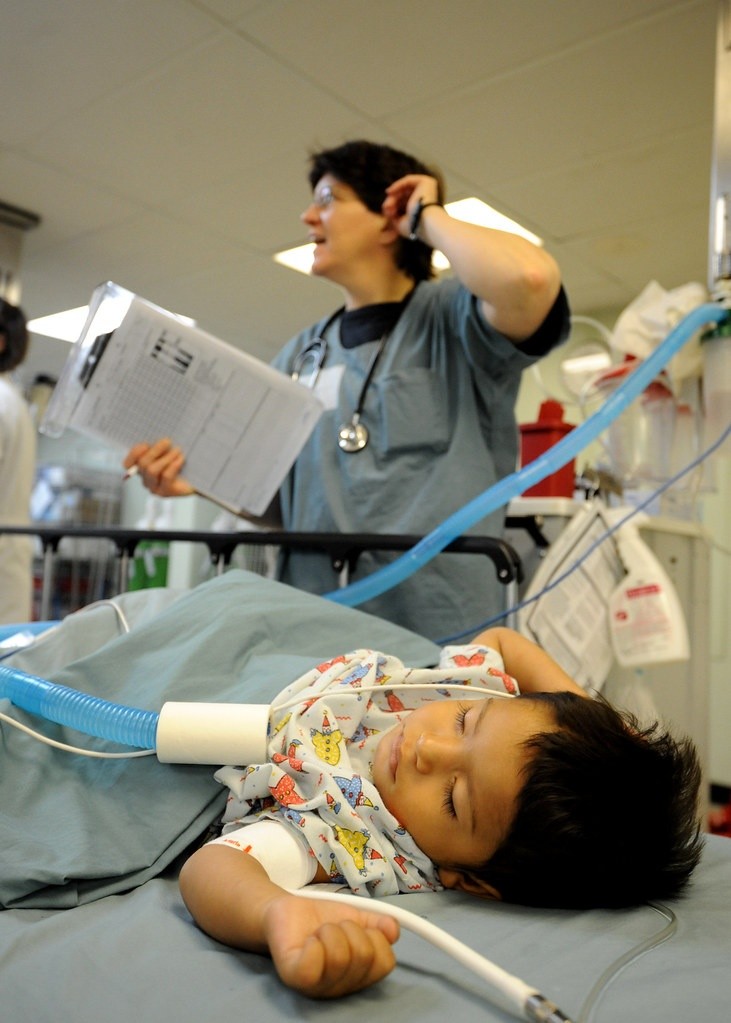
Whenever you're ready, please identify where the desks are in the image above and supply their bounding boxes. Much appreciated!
[506,493,713,833]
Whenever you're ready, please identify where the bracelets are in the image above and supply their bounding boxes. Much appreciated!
[408,197,443,244]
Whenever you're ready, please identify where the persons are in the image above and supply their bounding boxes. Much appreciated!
[177,625,703,1000]
[0,298,38,625]
[123,142,571,648]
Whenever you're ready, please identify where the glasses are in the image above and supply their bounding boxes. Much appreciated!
[308,186,376,207]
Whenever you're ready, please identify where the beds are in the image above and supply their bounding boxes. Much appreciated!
[0,525,731,1023]
